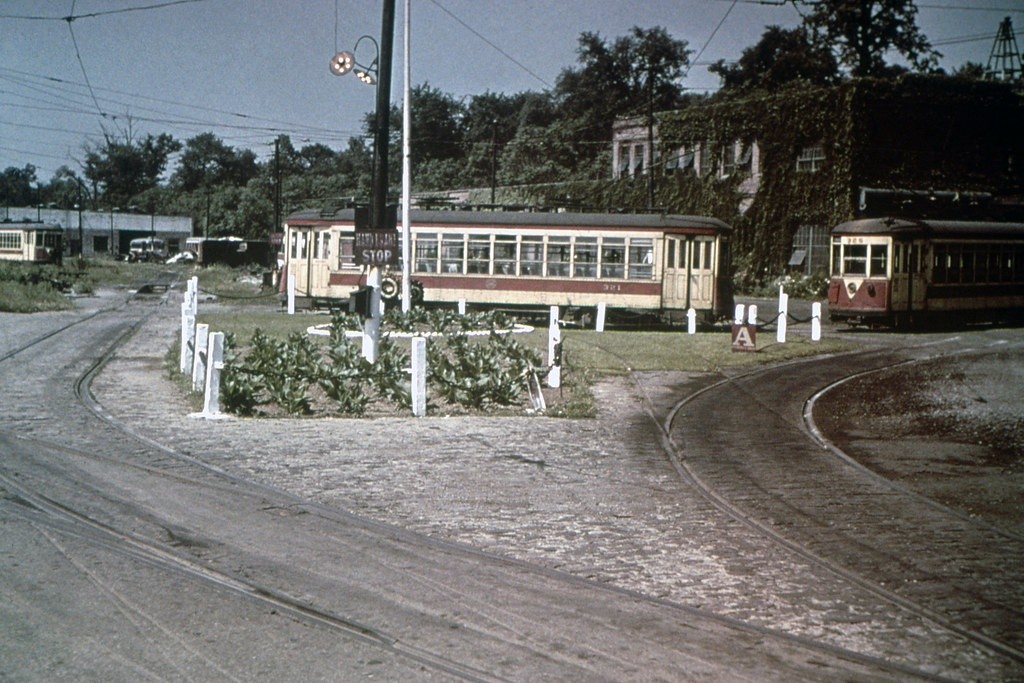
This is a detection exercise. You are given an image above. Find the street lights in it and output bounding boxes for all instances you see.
[330,1,403,367]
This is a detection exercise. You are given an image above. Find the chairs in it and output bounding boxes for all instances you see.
[467,264,622,278]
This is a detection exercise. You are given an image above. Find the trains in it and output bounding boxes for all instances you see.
[0,218,64,266]
[274,210,730,329]
[827,211,1022,330]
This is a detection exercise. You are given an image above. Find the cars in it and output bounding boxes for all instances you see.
[130,238,169,258]
